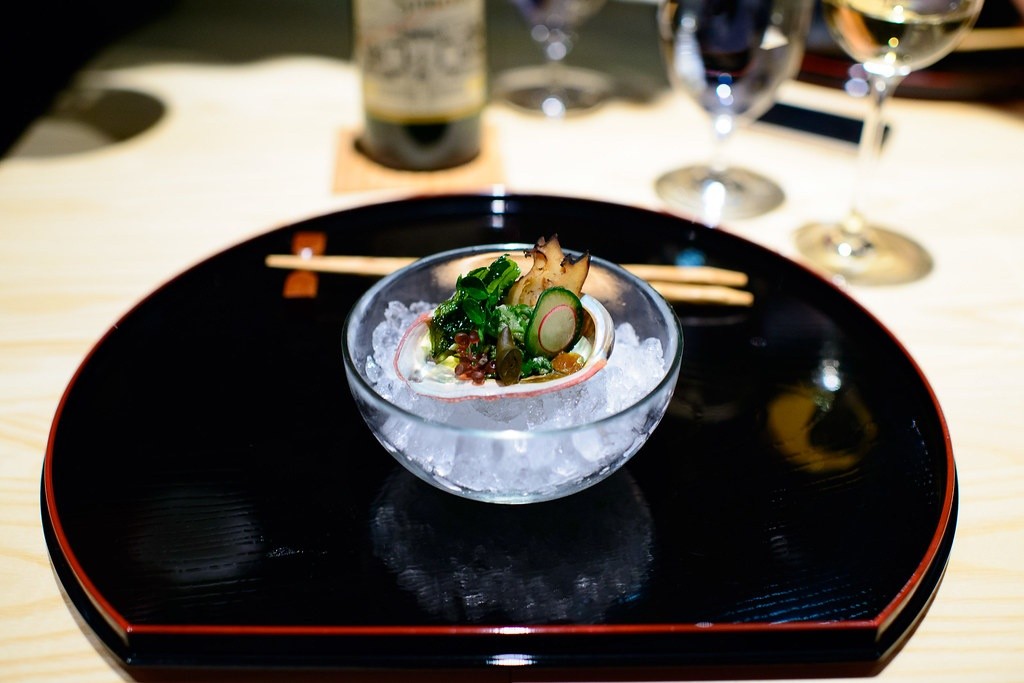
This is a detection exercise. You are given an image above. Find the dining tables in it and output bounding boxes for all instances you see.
[0,23,1024,683]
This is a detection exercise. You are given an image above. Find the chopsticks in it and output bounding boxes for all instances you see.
[265,254,755,306]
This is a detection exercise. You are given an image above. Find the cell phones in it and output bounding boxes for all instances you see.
[751,99,892,162]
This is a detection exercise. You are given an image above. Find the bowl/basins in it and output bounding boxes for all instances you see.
[341,244,683,503]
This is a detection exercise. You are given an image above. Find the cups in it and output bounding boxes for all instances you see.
[349,0,493,170]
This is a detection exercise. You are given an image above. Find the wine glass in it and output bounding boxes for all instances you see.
[650,0,812,218]
[514,0,606,116]
[791,0,986,290]
